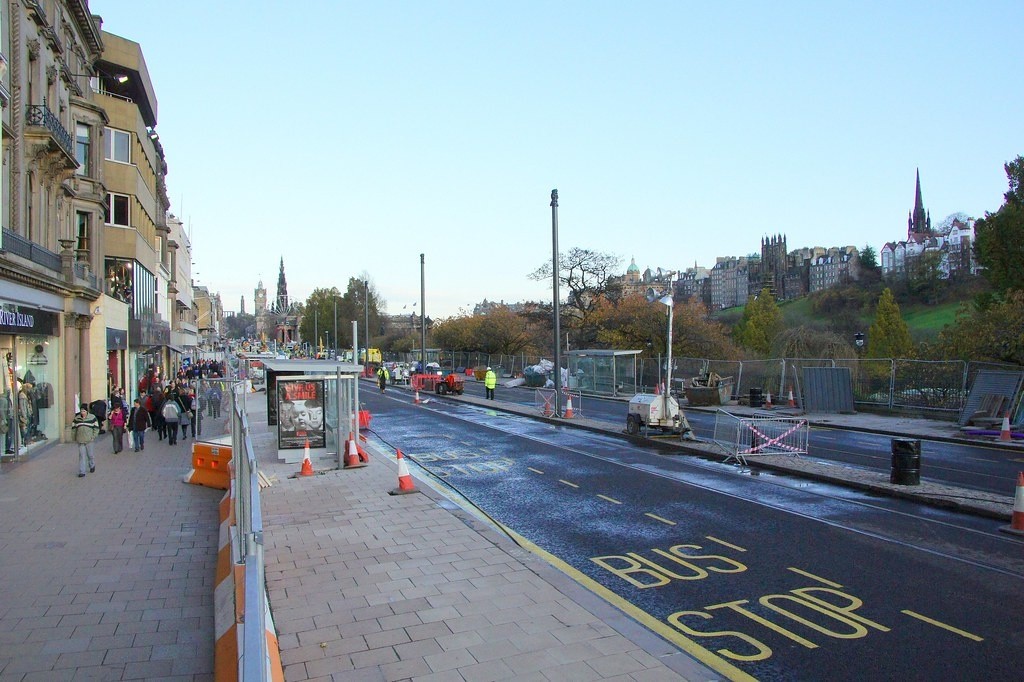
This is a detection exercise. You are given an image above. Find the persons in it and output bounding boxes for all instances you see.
[109,404,127,454]
[0,388,14,454]
[113,287,123,301]
[18,383,39,445]
[485,367,496,400]
[279,400,323,430]
[111,357,226,445]
[72,403,99,477]
[127,399,151,452]
[377,368,389,394]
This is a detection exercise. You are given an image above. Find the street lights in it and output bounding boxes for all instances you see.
[854,331,867,393]
[643,268,674,418]
[325,331,329,351]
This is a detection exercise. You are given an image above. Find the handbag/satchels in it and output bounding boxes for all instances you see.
[108,425,114,431]
[127,431,133,449]
[376,381,380,387]
[186,411,193,419]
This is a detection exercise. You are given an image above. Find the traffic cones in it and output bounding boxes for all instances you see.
[562,394,575,418]
[345,432,368,468]
[661,377,666,391]
[995,411,1016,443]
[562,378,568,392]
[295,438,319,479]
[761,391,774,410]
[654,384,659,394]
[785,386,798,408]
[997,472,1024,538]
[392,448,421,495]
[413,387,423,404]
[543,396,554,416]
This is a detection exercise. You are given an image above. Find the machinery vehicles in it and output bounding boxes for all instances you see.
[435,374,464,395]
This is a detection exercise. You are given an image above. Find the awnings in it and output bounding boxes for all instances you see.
[142,345,162,355]
[166,345,185,354]
[197,347,206,353]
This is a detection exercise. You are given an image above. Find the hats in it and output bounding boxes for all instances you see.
[487,367,491,370]
[80,404,87,411]
[382,367,386,371]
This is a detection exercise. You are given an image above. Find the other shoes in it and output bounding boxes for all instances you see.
[79,474,85,477]
[381,389,385,394]
[90,466,95,473]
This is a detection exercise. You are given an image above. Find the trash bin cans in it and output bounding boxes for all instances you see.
[891,437,921,485]
[473,364,505,380]
[524,370,555,386]
[750,388,762,407]
